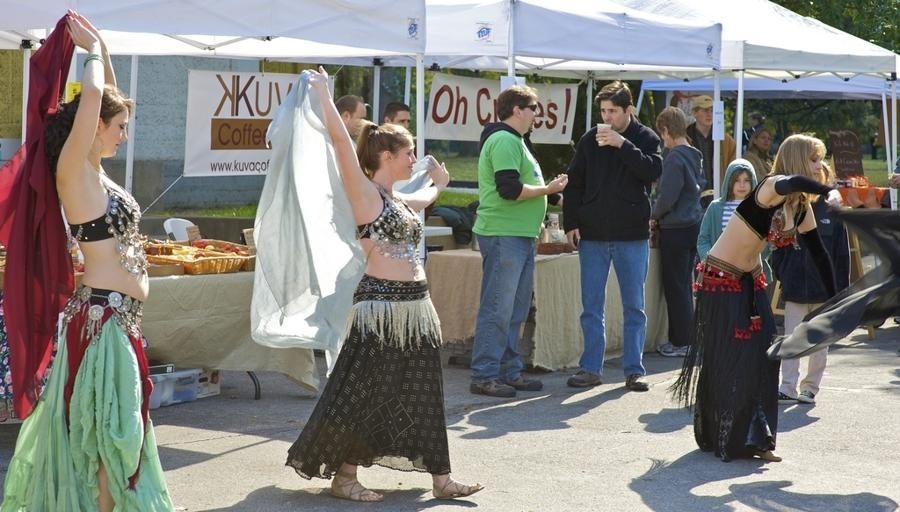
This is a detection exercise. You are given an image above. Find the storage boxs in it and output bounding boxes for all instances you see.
[197,367,221,399]
[149,366,203,411]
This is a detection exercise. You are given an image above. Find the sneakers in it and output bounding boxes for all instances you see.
[798,392,815,403]
[472,376,516,398]
[626,375,648,391]
[503,373,544,391]
[655,341,692,357]
[777,393,797,405]
[567,371,601,387]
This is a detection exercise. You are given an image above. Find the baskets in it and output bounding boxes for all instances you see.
[145,239,257,276]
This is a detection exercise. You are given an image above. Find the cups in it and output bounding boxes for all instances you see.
[541,213,561,244]
[596,123,613,144]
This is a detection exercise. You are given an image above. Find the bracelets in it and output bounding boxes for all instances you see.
[84,54,105,67]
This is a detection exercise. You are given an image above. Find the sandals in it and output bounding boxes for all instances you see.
[331,469,383,503]
[434,477,482,501]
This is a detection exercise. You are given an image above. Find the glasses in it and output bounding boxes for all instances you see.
[520,103,536,112]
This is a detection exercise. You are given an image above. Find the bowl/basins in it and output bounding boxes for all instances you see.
[839,185,884,206]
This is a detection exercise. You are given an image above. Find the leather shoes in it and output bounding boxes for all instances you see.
[758,451,780,461]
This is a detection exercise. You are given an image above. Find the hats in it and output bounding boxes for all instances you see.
[692,96,715,108]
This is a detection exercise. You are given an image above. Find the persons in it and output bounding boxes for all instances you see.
[0,9,173,512]
[697,158,773,285]
[650,107,703,357]
[888,158,900,188]
[871,133,878,159]
[524,132,564,225]
[334,95,367,150]
[771,162,850,404]
[743,125,773,183]
[562,80,663,391]
[470,85,569,397]
[285,65,486,502]
[685,95,736,208]
[383,102,411,130]
[665,133,843,461]
[742,112,765,150]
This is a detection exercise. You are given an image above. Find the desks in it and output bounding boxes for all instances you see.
[426,247,669,373]
[142,270,318,401]
